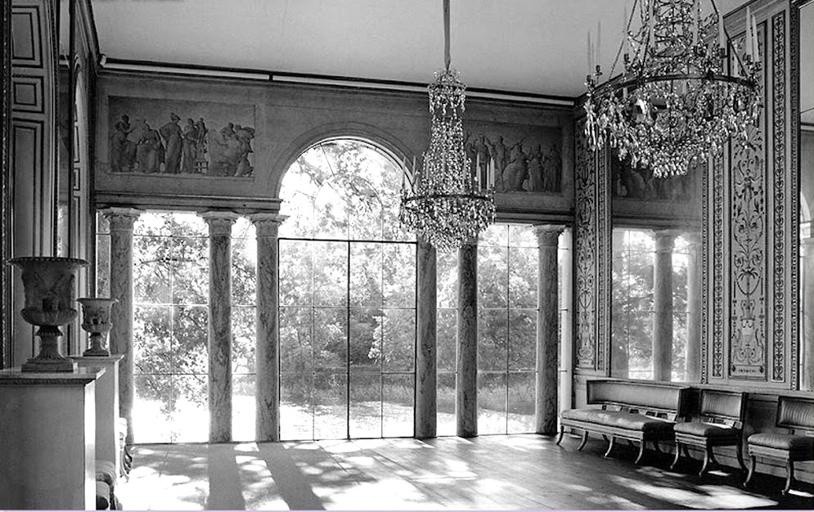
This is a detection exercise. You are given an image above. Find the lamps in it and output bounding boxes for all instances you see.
[396,1,499,255]
[581,2,765,182]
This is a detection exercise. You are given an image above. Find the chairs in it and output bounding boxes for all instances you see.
[743,394,814,495]
[668,387,750,479]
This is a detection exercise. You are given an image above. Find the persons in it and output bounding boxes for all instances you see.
[110,110,257,178]
[462,125,565,194]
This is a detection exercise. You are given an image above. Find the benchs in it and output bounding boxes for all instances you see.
[556,379,696,467]
